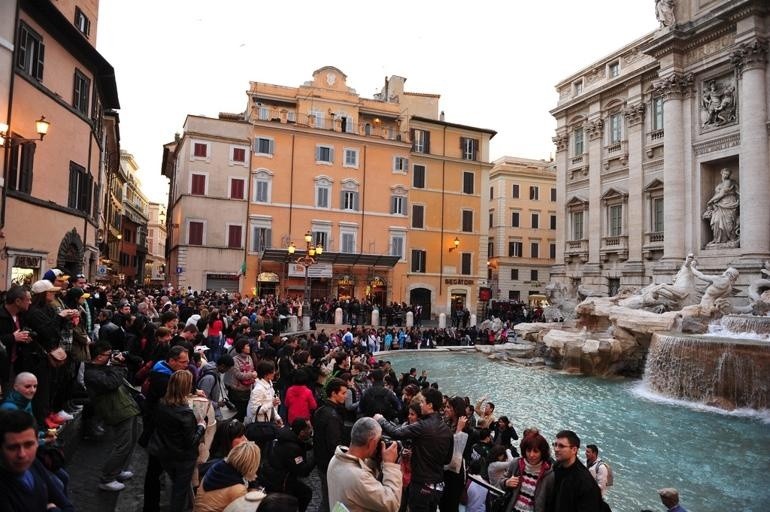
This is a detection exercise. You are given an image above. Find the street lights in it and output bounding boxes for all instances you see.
[0,114,50,149]
[286,228,324,320]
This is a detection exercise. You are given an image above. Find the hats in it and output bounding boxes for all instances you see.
[30,279,61,295]
[68,285,91,300]
[656,486,681,500]
[43,266,69,282]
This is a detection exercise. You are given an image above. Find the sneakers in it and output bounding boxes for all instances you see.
[98,479,128,492]
[113,470,134,481]
[41,401,83,433]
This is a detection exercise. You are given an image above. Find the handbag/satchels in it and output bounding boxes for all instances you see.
[242,402,282,442]
[46,347,68,368]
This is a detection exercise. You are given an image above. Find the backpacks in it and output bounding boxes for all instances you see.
[595,459,615,488]
[235,354,256,387]
[320,369,343,404]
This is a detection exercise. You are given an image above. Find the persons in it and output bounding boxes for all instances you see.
[714,86,735,125]
[667,262,738,315]
[705,166,740,242]
[701,82,724,125]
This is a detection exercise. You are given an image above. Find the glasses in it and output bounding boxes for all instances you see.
[75,274,86,278]
[552,441,576,449]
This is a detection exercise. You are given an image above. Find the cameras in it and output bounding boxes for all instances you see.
[112,350,128,360]
[221,397,236,410]
[377,438,403,453]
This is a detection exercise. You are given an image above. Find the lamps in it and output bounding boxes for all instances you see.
[448,236,461,253]
[1,113,51,149]
